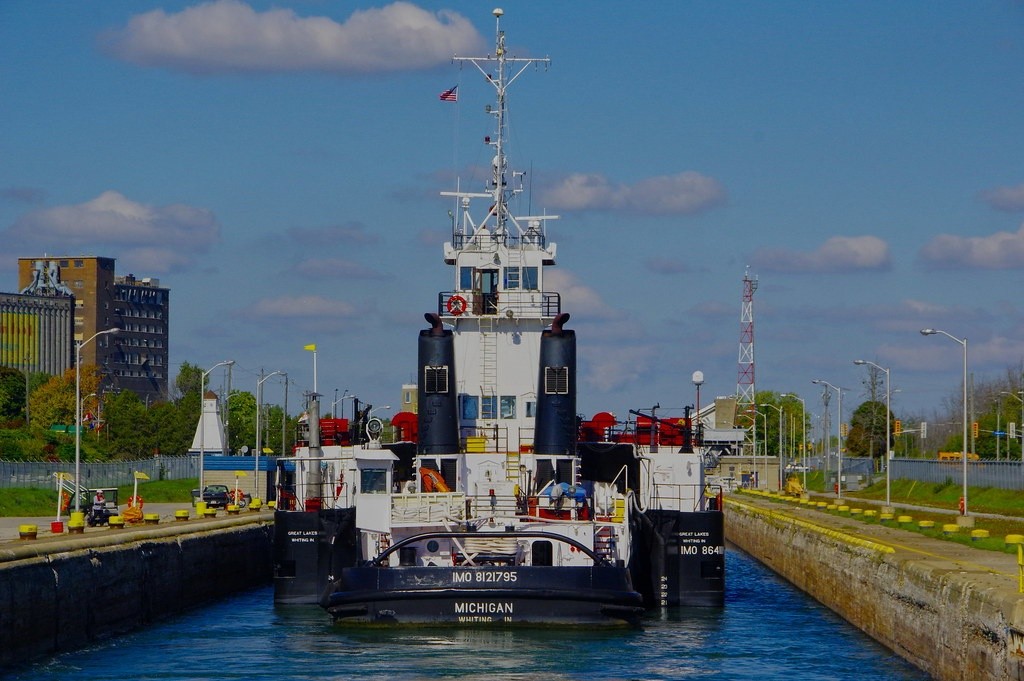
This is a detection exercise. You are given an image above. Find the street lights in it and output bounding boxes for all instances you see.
[196,361,235,512]
[370,406,391,420]
[332,395,356,419]
[920,328,975,528]
[737,394,810,500]
[71,328,120,520]
[222,394,238,426]
[812,380,845,505]
[1001,392,1024,461]
[853,359,894,514]
[81,394,96,425]
[880,390,902,472]
[252,372,288,505]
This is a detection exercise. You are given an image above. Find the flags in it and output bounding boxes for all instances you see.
[440,86,457,102]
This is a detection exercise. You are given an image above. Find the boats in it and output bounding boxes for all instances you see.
[272,8,725,628]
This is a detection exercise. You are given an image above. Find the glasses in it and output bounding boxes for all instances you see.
[99,493,102,494]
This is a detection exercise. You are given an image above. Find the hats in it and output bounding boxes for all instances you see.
[97,490,103,493]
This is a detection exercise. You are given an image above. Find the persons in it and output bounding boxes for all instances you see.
[89,490,108,527]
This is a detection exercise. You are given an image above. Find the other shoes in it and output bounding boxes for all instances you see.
[101,525,106,527]
[87,524,91,528]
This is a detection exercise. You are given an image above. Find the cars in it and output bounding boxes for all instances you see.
[203,484,231,509]
[784,463,809,472]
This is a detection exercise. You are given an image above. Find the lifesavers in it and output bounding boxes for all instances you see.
[230,488,244,501]
[60,490,70,510]
[959,497,964,515]
[447,295,467,315]
[127,495,144,510]
[835,482,838,494]
